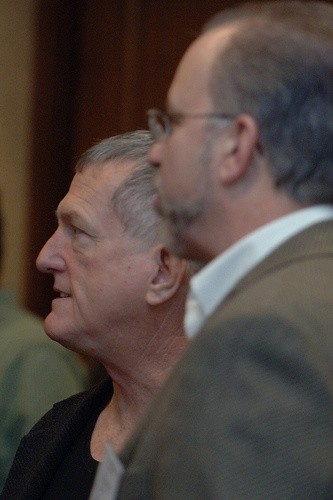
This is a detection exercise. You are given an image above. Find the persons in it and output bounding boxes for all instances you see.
[94,2,332,500]
[0,132,208,499]
[0,214,90,497]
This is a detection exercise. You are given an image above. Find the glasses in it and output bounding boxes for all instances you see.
[145,110,266,158]
